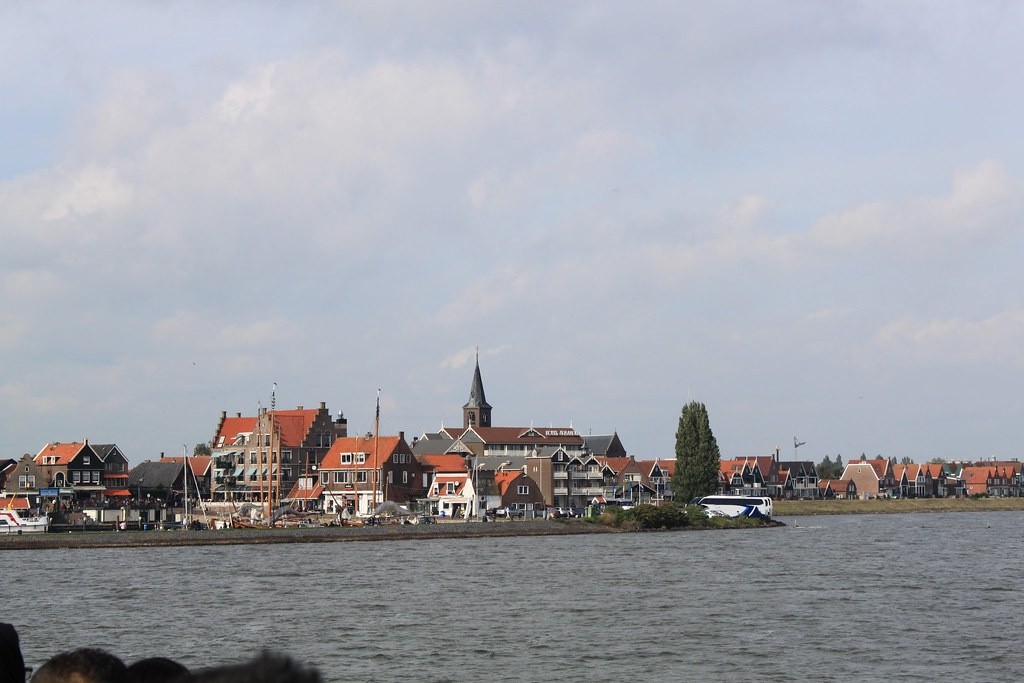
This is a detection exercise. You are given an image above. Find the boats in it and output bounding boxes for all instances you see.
[0,493,53,534]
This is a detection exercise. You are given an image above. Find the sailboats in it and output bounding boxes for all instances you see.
[230,383,381,529]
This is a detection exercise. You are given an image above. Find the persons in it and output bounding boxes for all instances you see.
[119,521,127,531]
[0,622,325,683]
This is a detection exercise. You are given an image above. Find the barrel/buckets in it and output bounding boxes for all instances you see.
[441,511,445,517]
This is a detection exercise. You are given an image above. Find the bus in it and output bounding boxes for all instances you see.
[689,493,773,519]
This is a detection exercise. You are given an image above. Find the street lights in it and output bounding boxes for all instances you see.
[475,454,486,523]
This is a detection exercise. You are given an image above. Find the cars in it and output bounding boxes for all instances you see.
[554,506,585,518]
[486,505,524,518]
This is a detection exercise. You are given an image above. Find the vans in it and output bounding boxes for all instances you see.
[604,496,637,511]
